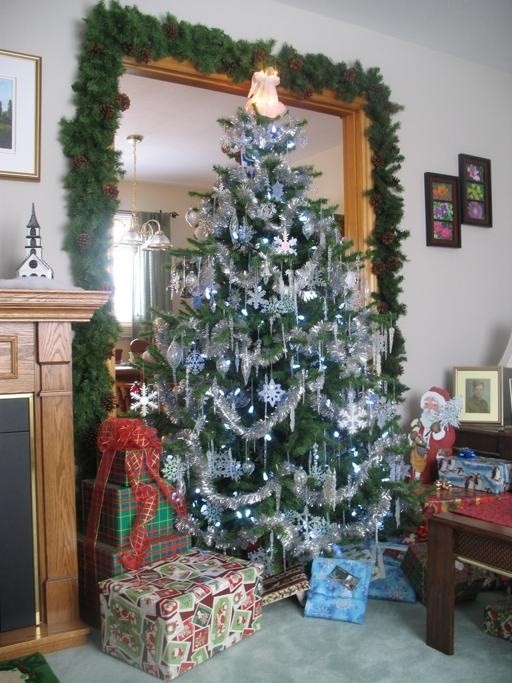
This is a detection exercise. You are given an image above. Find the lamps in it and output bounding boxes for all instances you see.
[244,66,288,119]
[109,134,174,252]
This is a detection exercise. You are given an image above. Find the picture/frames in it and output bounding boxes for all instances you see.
[424,151,491,247]
[455,365,512,425]
[0,49,44,181]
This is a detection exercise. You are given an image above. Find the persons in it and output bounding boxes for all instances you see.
[465,378,491,410]
[406,385,457,481]
